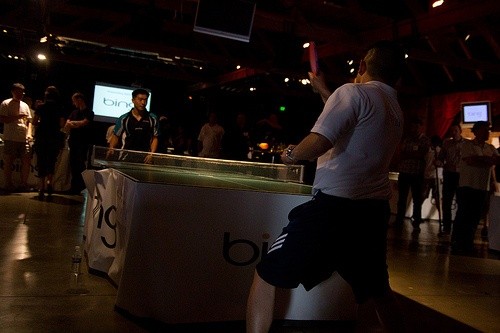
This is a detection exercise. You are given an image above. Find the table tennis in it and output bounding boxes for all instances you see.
[260,142,268,148]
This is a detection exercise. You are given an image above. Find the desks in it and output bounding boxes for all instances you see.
[388,171,485,224]
[81,158,363,332]
[0,143,73,191]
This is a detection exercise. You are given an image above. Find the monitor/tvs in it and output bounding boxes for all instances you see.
[91,81,153,124]
[460,101,492,128]
[194,0,257,44]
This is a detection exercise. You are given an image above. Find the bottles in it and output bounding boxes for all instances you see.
[71,246,81,274]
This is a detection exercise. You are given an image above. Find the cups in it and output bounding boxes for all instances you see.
[22,112,28,121]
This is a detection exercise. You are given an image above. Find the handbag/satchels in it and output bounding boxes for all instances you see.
[31,124,45,153]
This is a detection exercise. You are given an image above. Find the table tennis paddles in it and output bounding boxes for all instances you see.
[308,41,319,94]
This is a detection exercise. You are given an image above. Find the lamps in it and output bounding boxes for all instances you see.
[37,43,50,60]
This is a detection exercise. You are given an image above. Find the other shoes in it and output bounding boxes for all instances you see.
[481,228,489,241]
[442,227,452,233]
[408,215,421,233]
[46,196,53,202]
[35,193,44,201]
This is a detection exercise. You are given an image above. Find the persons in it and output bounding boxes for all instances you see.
[31,85,65,202]
[65,92,96,195]
[392,115,429,235]
[448,121,499,257]
[220,112,251,159]
[432,123,466,236]
[0,82,33,192]
[196,113,225,158]
[245,38,406,333]
[24,94,43,129]
[425,138,445,230]
[104,88,159,164]
[53,113,77,185]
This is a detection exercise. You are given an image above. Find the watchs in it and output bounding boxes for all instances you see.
[286,146,298,163]
